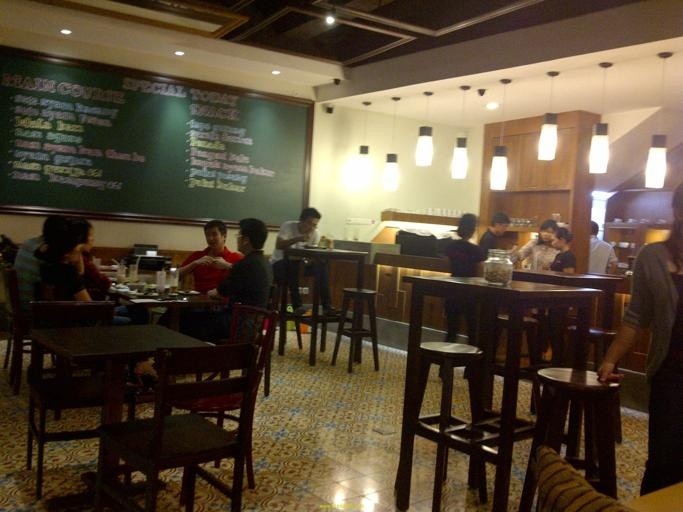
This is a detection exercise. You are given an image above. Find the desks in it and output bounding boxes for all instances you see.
[277,247,369,367]
[394,275,603,512]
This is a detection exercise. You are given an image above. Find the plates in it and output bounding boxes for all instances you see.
[135,292,159,297]
[179,290,201,295]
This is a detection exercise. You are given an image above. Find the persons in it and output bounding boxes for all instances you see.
[588,221,617,274]
[41,222,91,302]
[15,215,65,310]
[0,234,18,265]
[551,227,576,273]
[155,219,244,325]
[479,212,511,252]
[271,208,342,316]
[438,213,488,379]
[67,217,111,301]
[597,183,683,496]
[509,219,561,271]
[180,218,273,342]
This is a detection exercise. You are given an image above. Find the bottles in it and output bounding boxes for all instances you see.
[483,249,512,287]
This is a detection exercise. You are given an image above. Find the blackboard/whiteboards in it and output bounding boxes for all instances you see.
[0,44,316,231]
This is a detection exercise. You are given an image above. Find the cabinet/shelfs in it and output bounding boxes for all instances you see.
[478,111,599,275]
[372,209,464,244]
[601,189,679,278]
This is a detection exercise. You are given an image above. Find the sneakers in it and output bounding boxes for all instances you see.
[323,307,342,316]
[295,307,306,317]
[126,381,138,388]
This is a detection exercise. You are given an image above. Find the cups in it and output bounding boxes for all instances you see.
[116,264,127,283]
[156,270,169,299]
[130,263,139,284]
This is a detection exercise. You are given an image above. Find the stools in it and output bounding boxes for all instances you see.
[395,339,487,512]
[331,287,379,374]
[495,315,543,415]
[563,326,618,471]
[518,365,621,510]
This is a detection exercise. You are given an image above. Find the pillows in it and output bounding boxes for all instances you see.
[528,446,638,512]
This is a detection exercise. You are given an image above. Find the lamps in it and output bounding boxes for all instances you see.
[645,52,674,189]
[589,63,613,175]
[450,85,470,181]
[490,78,511,192]
[536,71,561,161]
[343,101,373,192]
[415,90,434,167]
[381,96,401,196]
